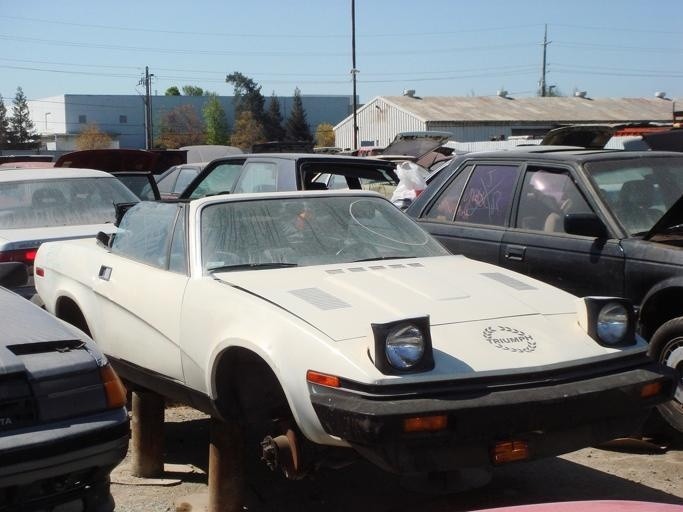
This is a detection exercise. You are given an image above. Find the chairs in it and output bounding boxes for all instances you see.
[32,188,66,209]
[618,179,664,232]
[306,181,329,191]
